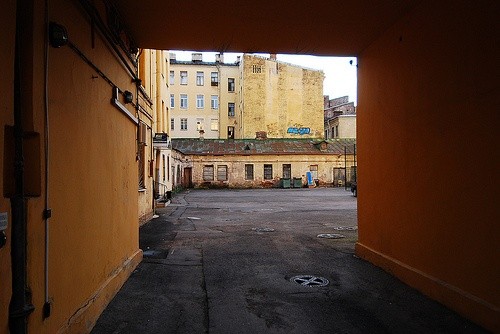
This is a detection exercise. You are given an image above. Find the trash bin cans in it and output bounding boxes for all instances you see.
[293,176,303,188]
[280,177,291,189]
